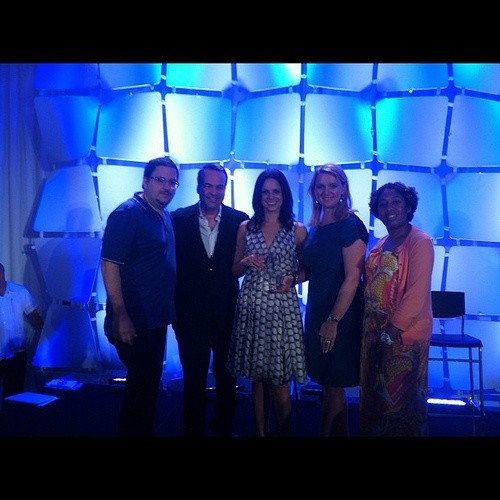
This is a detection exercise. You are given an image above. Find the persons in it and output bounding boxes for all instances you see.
[357,181,434,436]
[0,262,50,395]
[101,157,179,436]
[231,168,308,436]
[174,161,250,436]
[297,163,370,436]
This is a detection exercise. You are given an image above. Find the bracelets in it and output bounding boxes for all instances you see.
[287,271,298,288]
[328,314,341,325]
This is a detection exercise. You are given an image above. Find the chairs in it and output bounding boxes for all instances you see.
[424,291,484,410]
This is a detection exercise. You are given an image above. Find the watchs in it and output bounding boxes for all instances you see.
[379,330,393,346]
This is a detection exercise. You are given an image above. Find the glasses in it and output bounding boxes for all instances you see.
[148,175,179,189]
